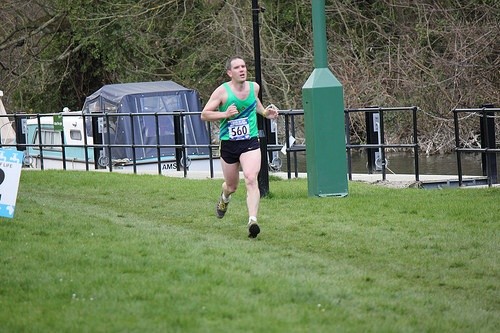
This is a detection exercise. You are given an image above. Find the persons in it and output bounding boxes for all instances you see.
[200,56,279,239]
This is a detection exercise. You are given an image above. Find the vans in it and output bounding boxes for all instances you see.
[22,116,93,161]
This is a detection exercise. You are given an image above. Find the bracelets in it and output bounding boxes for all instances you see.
[262,109,267,117]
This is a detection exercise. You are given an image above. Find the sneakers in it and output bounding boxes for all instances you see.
[216,192,230,219]
[248,221,260,238]
